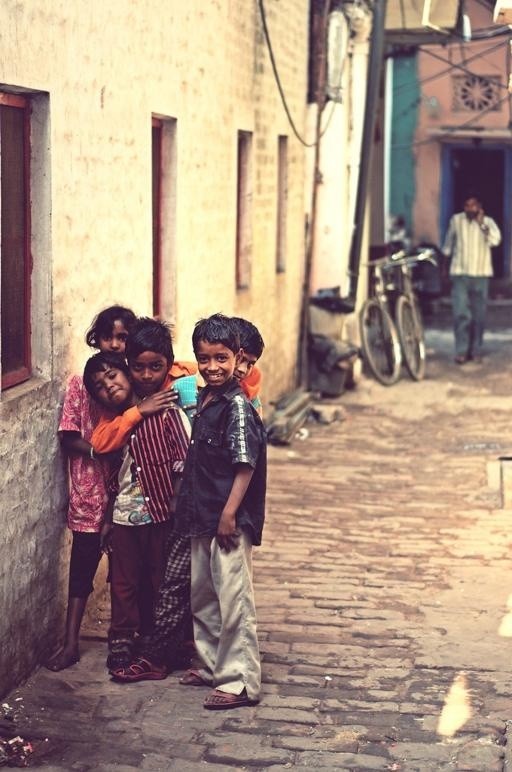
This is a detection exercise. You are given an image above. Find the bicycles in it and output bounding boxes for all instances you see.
[358,247,404,387]
[383,247,439,382]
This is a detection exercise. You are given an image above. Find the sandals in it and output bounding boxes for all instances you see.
[106,637,258,709]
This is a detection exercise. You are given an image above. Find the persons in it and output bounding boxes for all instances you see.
[79,350,193,670]
[171,312,271,711]
[434,190,506,366]
[44,305,138,675]
